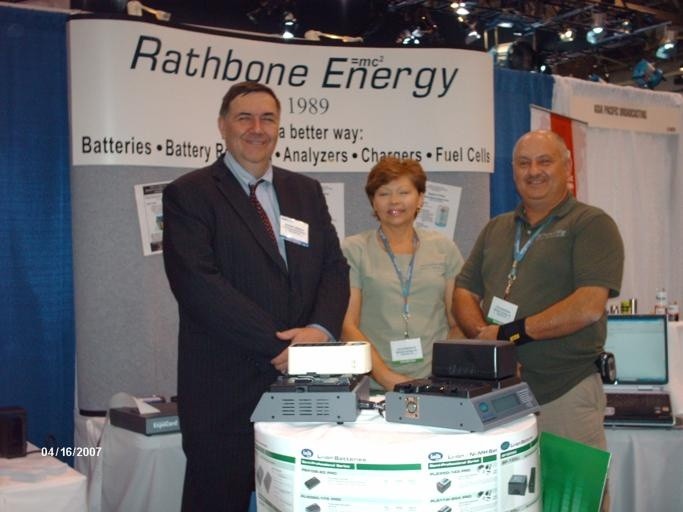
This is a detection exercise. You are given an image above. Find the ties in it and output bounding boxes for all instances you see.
[248,180,283,261]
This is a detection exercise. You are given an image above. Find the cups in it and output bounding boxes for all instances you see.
[609,296,637,316]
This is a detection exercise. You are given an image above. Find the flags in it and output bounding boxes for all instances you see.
[530,105,588,206]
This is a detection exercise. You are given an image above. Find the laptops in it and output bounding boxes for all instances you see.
[602,315,675,427]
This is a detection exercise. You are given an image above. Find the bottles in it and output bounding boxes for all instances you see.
[653,286,680,322]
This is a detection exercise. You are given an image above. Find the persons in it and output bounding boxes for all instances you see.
[341,156,465,397]
[161,80,352,512]
[449,129,625,512]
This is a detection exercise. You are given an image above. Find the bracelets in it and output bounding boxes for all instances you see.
[497,317,534,347]
